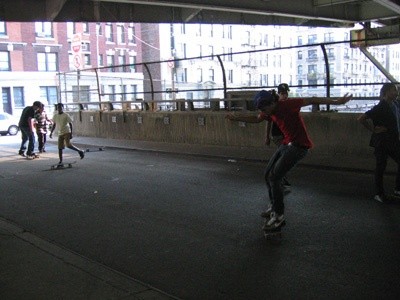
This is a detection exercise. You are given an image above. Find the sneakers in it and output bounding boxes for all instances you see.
[27,153,35,156]
[52,162,64,168]
[373,195,383,203]
[262,211,287,231]
[79,150,85,159]
[391,191,400,199]
[18,153,26,157]
[259,204,272,217]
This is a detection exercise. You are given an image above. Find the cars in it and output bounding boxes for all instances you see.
[0,113,20,136]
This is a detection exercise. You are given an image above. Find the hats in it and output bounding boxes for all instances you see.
[34,101,42,108]
[255,90,272,108]
[278,83,290,93]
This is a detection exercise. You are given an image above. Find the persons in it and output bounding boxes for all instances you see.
[19,101,41,157]
[356,82,400,203]
[266,83,292,186]
[49,103,85,165]
[225,90,354,231]
[33,104,53,152]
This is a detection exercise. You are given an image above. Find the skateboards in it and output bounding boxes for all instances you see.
[27,154,40,160]
[47,161,77,169]
[264,202,282,240]
[85,148,104,152]
